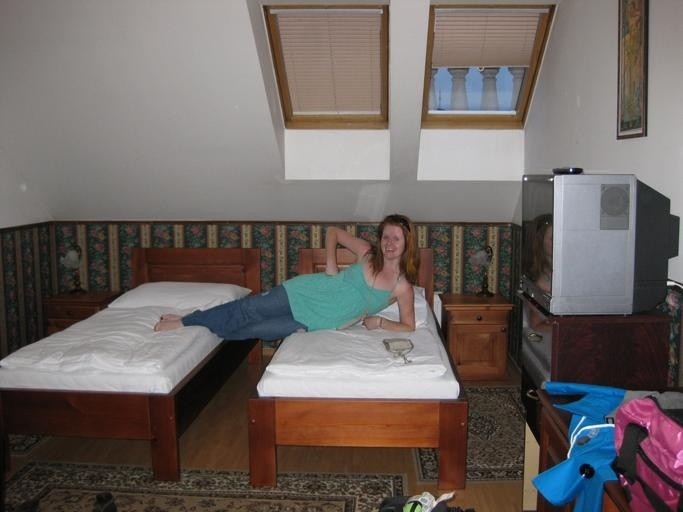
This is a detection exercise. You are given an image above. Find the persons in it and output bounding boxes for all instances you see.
[155,214,420,340]
[527,214,553,297]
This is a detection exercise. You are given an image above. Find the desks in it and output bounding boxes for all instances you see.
[535,386,682,512]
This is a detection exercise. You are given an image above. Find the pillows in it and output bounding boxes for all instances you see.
[371,280,430,328]
[109,281,252,311]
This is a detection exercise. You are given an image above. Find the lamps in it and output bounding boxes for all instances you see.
[60,244,89,298]
[475,243,493,297]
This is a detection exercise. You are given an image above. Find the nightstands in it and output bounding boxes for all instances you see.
[42,290,118,338]
[439,293,515,381]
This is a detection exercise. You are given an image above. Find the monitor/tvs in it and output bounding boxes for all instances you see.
[521,173,680,316]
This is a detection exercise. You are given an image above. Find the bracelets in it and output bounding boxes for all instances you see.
[381,317,384,330]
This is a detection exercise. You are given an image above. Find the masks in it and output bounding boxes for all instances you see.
[384,338,413,363]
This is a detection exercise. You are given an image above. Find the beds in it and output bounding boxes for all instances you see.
[1,248,261,481]
[245,246,467,488]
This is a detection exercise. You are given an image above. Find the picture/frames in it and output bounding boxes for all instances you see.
[616,16,648,140]
